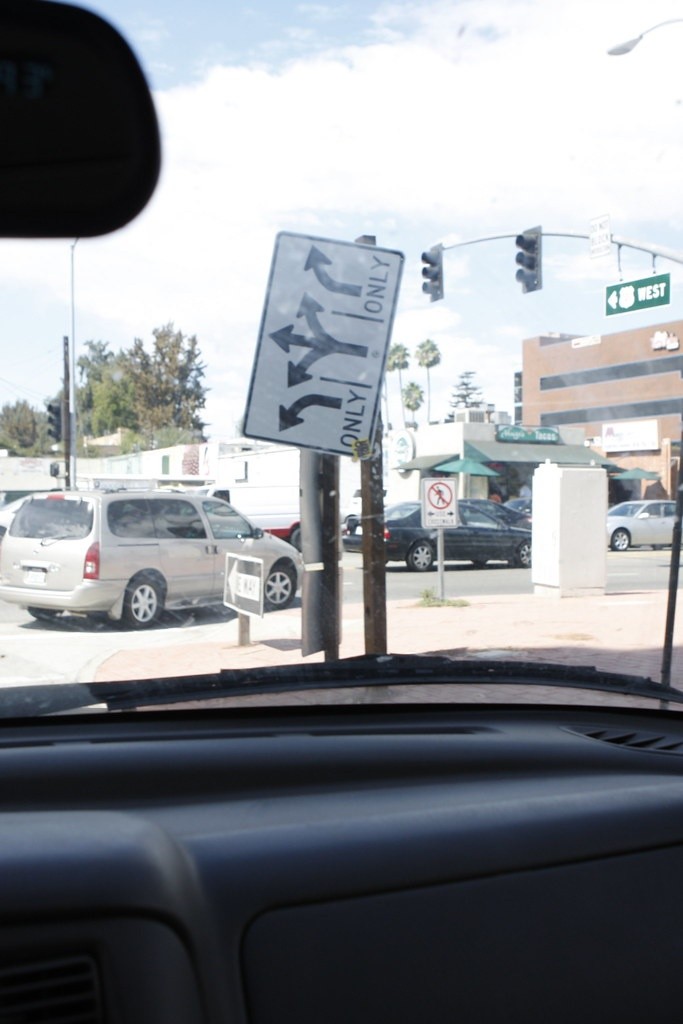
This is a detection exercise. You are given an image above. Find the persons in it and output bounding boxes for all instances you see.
[489,480,531,502]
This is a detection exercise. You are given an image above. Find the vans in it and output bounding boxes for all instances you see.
[161,482,302,553]
[0,489,304,630]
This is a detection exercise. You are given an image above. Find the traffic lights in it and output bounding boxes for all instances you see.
[421,243,444,301]
[516,226,543,293]
[47,404,61,442]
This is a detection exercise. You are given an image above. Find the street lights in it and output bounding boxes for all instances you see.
[607,17,683,56]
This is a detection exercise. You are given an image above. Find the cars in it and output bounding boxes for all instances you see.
[459,499,532,530]
[503,498,532,516]
[608,500,683,552]
[342,503,532,572]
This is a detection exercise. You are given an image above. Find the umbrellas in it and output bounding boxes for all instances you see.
[434,457,500,498]
[612,468,661,499]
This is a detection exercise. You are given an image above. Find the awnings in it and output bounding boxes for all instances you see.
[464,438,617,473]
[394,453,459,474]
